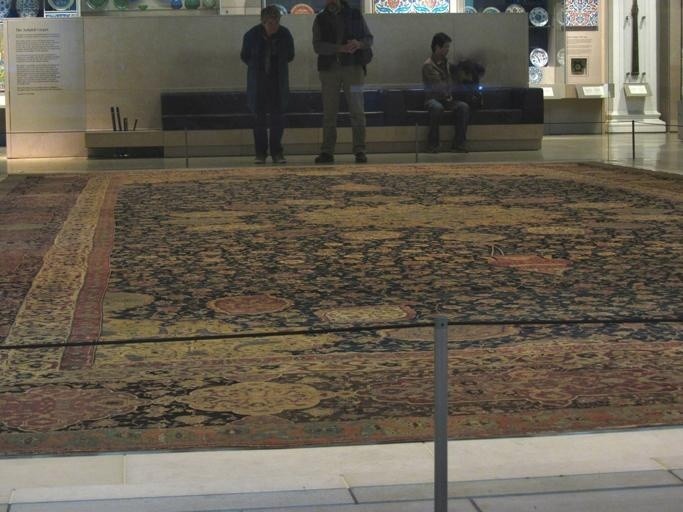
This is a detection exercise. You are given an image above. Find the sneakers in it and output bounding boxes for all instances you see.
[426,139,439,154]
[254,158,266,164]
[272,155,288,164]
[450,143,468,154]
[354,152,367,163]
[314,152,335,164]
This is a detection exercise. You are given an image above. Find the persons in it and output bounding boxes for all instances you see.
[311,0,374,163]
[420,33,472,154]
[238,7,294,164]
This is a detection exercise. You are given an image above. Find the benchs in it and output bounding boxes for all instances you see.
[157,88,544,149]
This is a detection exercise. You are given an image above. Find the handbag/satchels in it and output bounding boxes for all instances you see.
[353,46,373,66]
